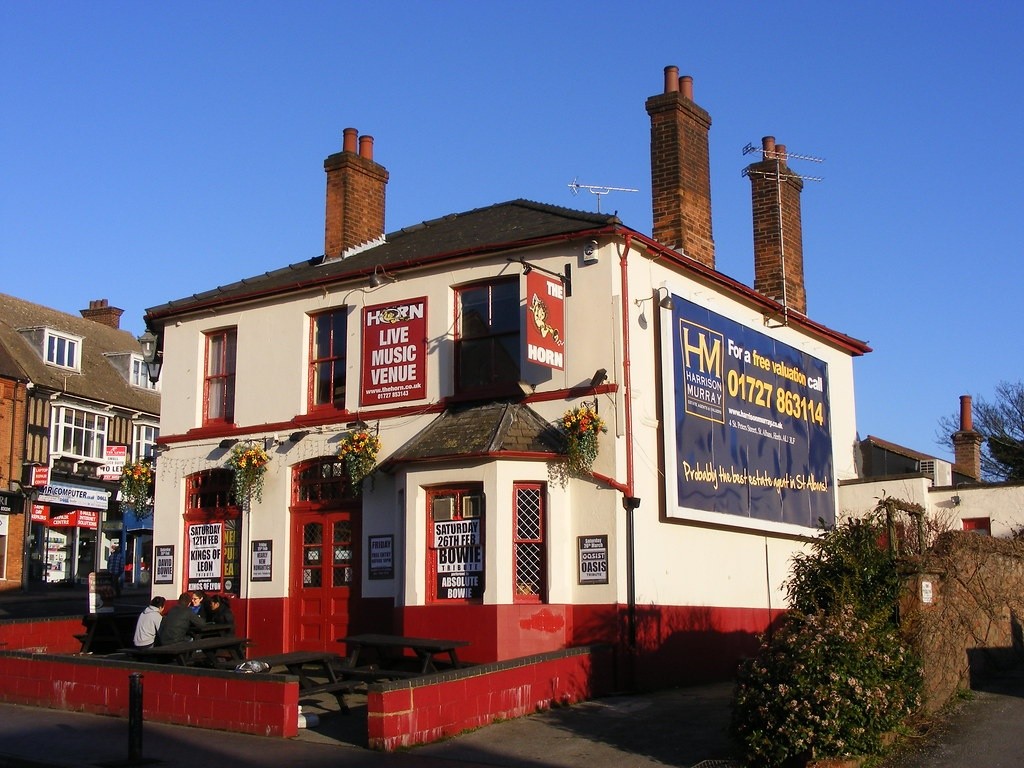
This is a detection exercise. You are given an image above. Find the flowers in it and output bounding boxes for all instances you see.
[336,430,382,500]
[550,407,609,492]
[223,444,273,515]
[118,460,155,523]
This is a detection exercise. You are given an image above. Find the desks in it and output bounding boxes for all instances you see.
[82,614,140,653]
[193,624,239,662]
[215,651,351,716]
[336,633,471,673]
[144,635,253,667]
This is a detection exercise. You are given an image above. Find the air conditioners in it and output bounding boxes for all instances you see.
[920,459,952,487]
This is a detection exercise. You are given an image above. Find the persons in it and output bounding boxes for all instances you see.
[107,546,124,599]
[133,596,166,650]
[189,591,207,614]
[201,594,235,662]
[160,592,206,666]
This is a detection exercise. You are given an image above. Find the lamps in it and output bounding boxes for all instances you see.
[348,421,368,430]
[142,351,163,385]
[219,439,239,449]
[289,431,306,442]
[369,263,398,289]
[137,327,158,363]
[635,286,673,310]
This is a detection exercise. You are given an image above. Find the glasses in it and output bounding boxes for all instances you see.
[191,598,199,601]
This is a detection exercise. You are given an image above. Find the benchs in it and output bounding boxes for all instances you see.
[117,648,146,656]
[300,678,364,698]
[333,667,424,680]
[385,656,481,669]
[80,633,134,644]
